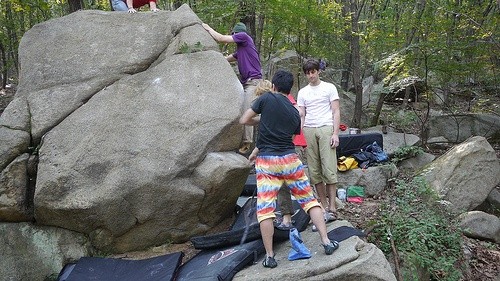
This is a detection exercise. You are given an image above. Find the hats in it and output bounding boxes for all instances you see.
[230,22,247,33]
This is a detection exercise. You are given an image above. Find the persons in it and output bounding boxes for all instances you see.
[200,20,263,155]
[110,0,160,14]
[238,70,339,268]
[297,60,339,221]
[318,57,330,71]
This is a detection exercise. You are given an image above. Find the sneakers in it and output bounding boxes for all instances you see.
[327,210,338,222]
[323,240,339,254]
[262,254,277,268]
[278,222,294,230]
[239,144,251,153]
